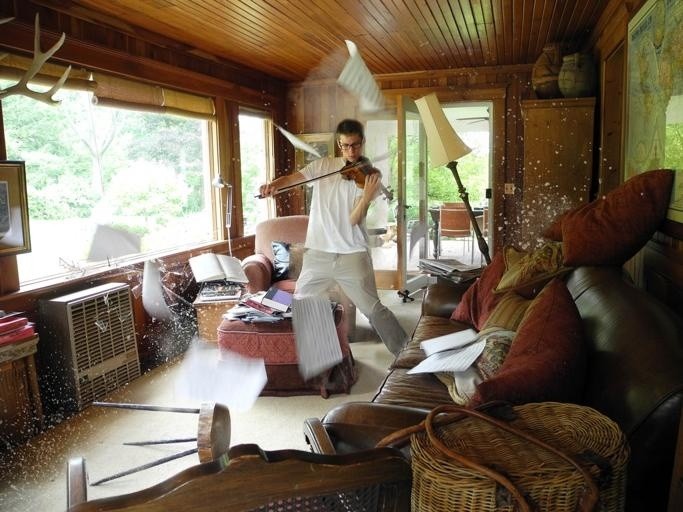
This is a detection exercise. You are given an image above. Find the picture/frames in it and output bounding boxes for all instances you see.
[2,161,33,255]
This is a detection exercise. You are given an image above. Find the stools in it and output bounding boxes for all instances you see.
[87,395,230,487]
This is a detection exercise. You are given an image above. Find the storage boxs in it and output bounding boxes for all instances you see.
[192,292,244,342]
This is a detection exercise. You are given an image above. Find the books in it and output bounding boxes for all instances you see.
[243,285,295,318]
[188,252,250,284]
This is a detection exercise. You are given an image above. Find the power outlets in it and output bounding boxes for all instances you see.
[505,184,515,194]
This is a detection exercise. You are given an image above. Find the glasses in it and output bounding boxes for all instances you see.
[338,140,362,150]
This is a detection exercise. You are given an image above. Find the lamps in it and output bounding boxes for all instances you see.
[212,174,232,257]
[414,92,490,263]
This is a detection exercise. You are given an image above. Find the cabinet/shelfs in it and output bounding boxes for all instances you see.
[0,334,49,438]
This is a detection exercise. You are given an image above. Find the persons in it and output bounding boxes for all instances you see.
[259,119,411,356]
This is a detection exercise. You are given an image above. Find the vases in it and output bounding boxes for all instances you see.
[531,46,560,99]
[560,53,592,96]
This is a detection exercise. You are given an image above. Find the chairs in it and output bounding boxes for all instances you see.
[64,416,409,512]
[443,202,470,252]
[472,209,489,266]
[438,208,473,257]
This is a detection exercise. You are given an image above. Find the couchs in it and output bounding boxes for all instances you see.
[238,216,355,342]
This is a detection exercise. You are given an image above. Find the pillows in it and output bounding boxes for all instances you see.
[470,327,515,380]
[559,169,677,266]
[481,294,533,331]
[322,267,682,512]
[450,252,504,329]
[503,245,526,266]
[479,278,583,405]
[492,240,569,291]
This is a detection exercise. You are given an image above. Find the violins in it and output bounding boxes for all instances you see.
[340,157,393,201]
